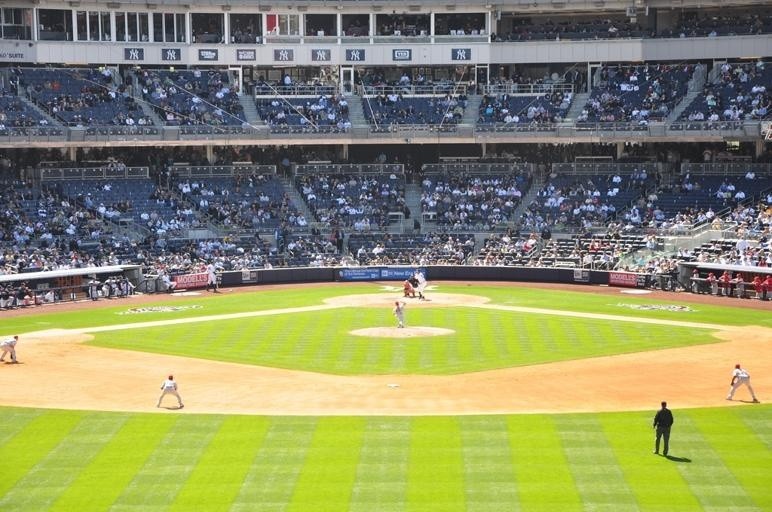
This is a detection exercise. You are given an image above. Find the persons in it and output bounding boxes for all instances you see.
[392,300,408,328]
[1,335,20,364]
[726,363,760,404]
[155,374,185,408]
[651,400,673,456]
[1,0,771,313]
